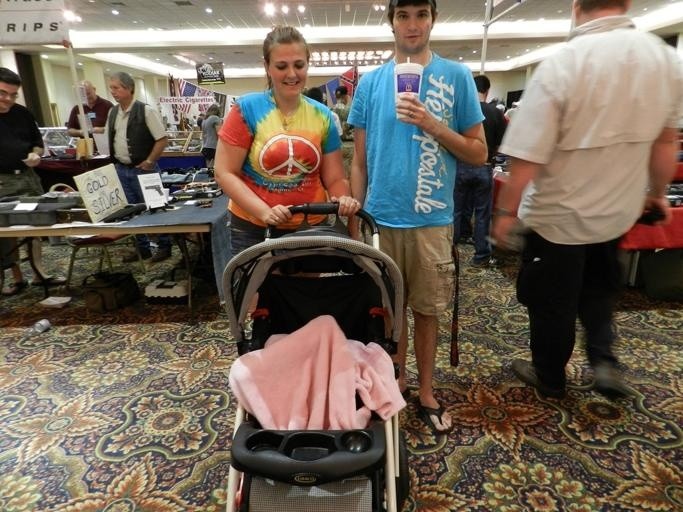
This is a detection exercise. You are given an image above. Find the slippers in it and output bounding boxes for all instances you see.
[31,274,66,285]
[414,394,453,434]
[1,278,27,296]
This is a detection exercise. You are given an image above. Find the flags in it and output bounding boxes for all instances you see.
[341,66,359,97]
[179,78,239,121]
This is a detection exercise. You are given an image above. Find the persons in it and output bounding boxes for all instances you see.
[454,74,523,270]
[64,78,116,138]
[102,72,173,264]
[210,26,360,317]
[343,2,489,438]
[308,85,354,179]
[177,104,222,169]
[0,66,68,296]
[489,0,683,400]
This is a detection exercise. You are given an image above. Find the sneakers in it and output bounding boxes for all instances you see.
[472,254,505,268]
[151,246,170,262]
[458,234,475,245]
[123,248,151,262]
[509,358,567,398]
[593,361,629,400]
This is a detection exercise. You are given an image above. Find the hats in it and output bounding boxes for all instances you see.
[335,86,346,95]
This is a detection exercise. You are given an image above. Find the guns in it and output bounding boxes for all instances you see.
[145,185,163,196]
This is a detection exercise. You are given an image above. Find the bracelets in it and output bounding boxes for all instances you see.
[146,160,156,168]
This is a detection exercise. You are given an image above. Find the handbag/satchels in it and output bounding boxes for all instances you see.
[81,271,140,314]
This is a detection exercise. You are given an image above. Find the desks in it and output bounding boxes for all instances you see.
[493,170,683,289]
[0,172,231,317]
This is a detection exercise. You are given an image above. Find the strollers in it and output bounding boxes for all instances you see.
[216,206,412,508]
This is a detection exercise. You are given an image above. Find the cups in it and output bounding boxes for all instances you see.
[393,63,424,119]
